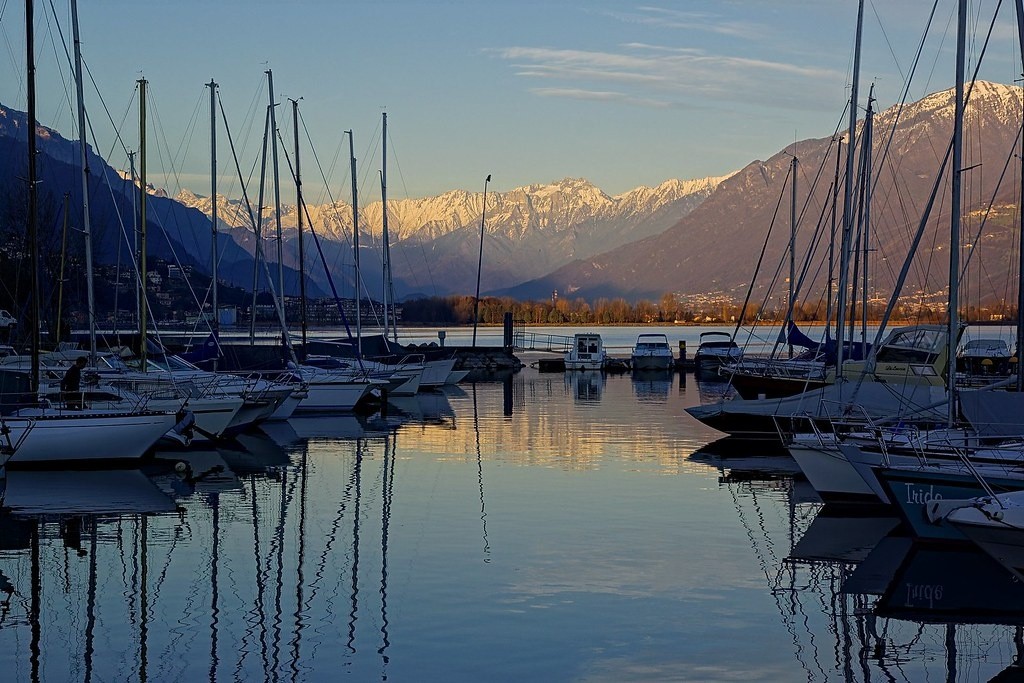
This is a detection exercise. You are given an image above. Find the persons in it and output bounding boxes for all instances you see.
[77,341,87,350]
[53,342,60,352]
[60,356,88,410]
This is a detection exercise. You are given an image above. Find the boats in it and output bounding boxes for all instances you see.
[695,332,745,370]
[630,333,675,371]
[564,331,605,371]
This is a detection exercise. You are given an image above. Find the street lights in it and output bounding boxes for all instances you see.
[472,173,492,349]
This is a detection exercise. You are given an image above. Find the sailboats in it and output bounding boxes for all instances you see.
[683,1,1023,588]
[0,1,470,464]
